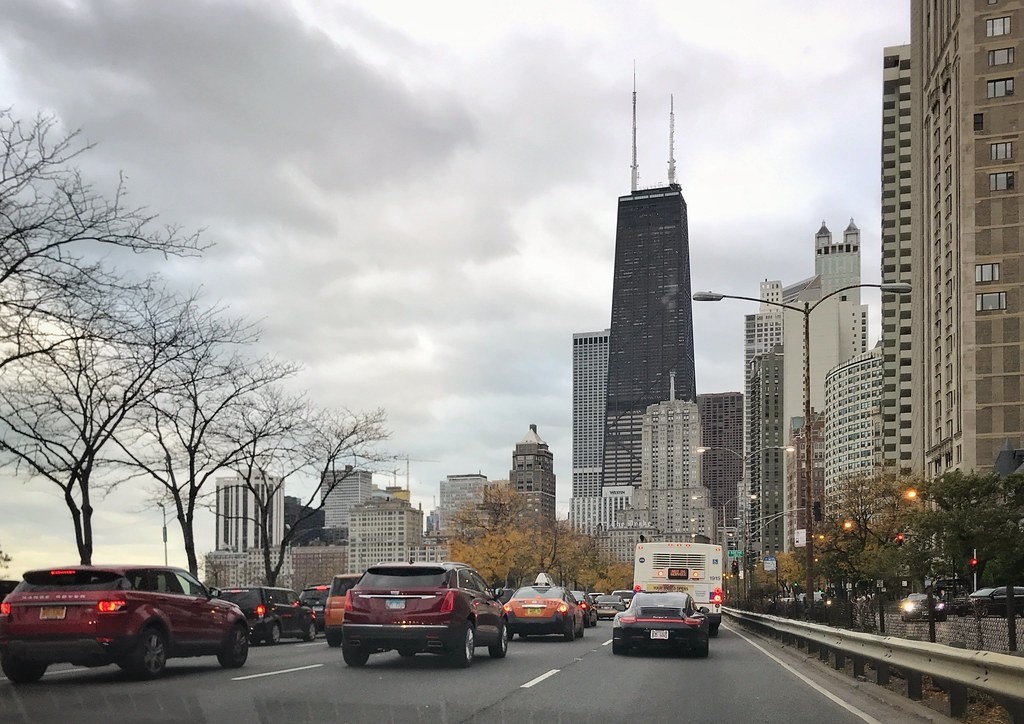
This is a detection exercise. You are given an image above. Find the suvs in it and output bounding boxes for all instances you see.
[950,586,1024,618]
[0,565,250,683]
[342,560,509,668]
[294,584,331,631]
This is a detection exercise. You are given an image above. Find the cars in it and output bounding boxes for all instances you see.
[492,588,515,604]
[611,590,635,609]
[218,587,318,646]
[570,591,598,627]
[612,589,709,658]
[595,596,629,620]
[502,573,585,641]
[900,594,947,622]
[323,574,364,647]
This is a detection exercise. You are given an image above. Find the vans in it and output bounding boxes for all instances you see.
[588,593,604,608]
[799,592,828,603]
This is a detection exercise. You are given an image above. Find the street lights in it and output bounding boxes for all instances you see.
[286,524,292,589]
[693,444,796,603]
[694,282,914,602]
[340,539,350,574]
[691,495,759,601]
[692,518,740,544]
[157,502,168,567]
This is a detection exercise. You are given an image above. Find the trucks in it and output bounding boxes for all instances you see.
[633,534,724,636]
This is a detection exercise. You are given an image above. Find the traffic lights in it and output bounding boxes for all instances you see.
[818,588,821,592]
[795,581,797,587]
[731,561,738,575]
[898,536,902,545]
[811,502,821,521]
[971,560,977,573]
